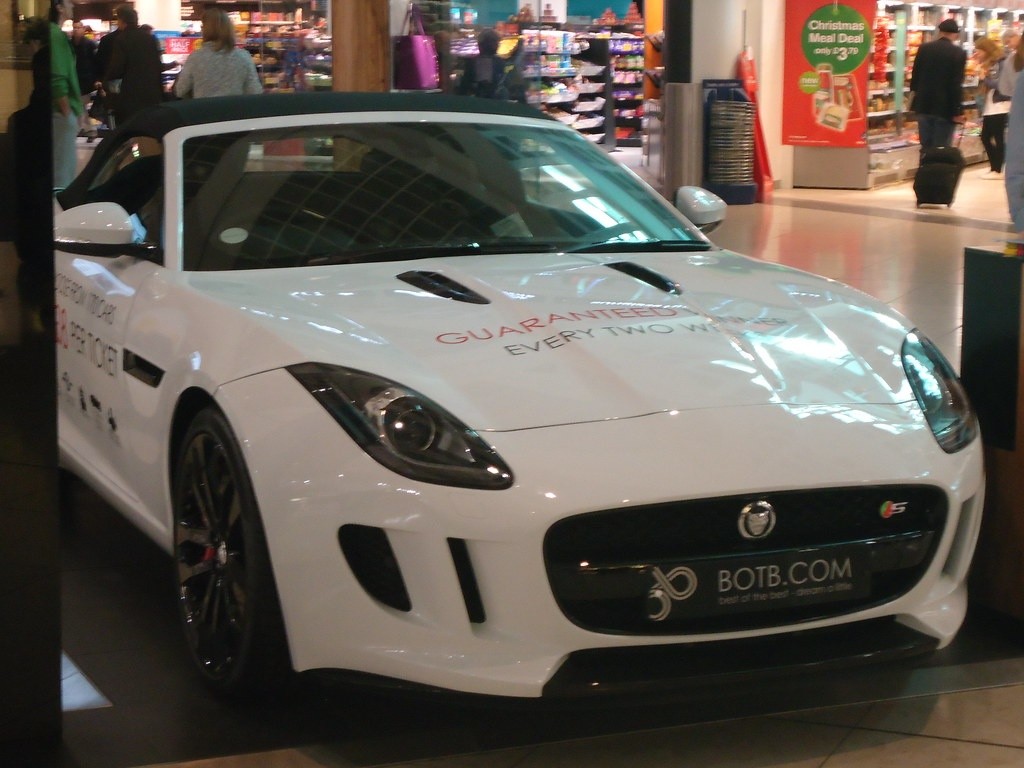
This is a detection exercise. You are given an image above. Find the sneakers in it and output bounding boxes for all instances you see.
[979,170,1004,180]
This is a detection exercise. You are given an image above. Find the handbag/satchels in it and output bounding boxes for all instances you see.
[392,5,439,89]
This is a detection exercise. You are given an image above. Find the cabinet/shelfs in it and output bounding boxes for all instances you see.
[791,3,1024,188]
[62,0,332,94]
[422,5,665,169]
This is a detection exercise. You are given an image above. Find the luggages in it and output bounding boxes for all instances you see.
[912,117,965,208]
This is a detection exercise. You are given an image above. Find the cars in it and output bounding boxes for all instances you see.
[54,89,988,723]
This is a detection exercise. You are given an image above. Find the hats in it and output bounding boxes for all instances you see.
[939,18,959,33]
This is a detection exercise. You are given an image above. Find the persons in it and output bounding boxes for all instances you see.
[971,36,1013,181]
[997,24,1024,238]
[0,0,169,207]
[172,8,264,100]
[457,29,507,97]
[906,18,967,161]
